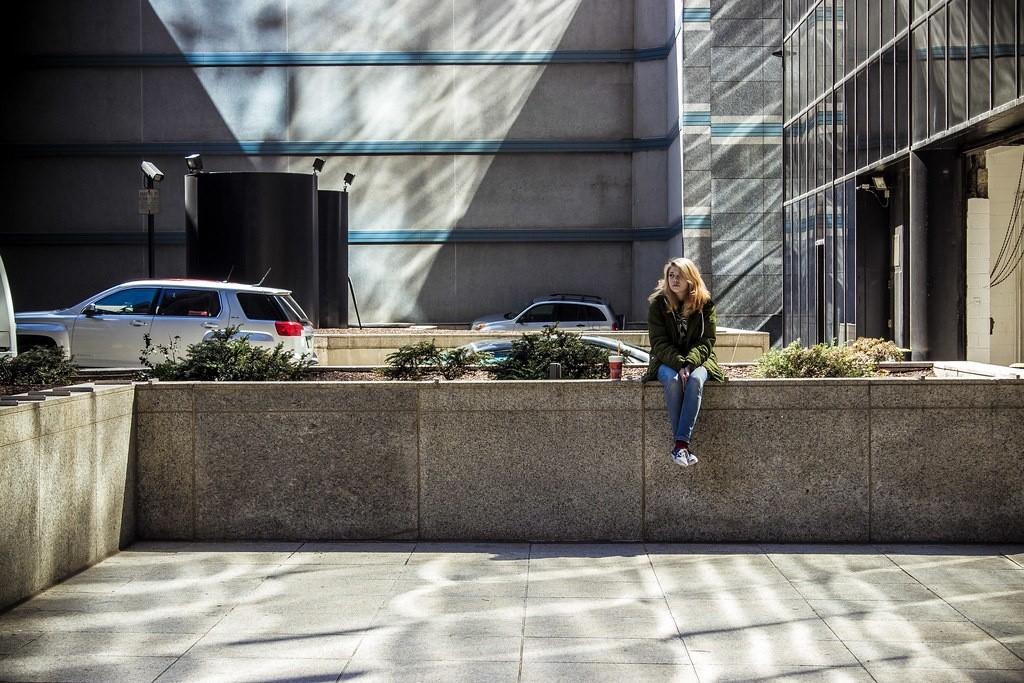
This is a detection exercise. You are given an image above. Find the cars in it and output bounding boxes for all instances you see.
[425,335,651,365]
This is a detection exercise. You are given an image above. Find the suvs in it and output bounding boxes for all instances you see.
[14,278,319,370]
[468,292,627,330]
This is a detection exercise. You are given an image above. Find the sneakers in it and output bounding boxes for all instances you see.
[686,454,698,465]
[671,448,689,467]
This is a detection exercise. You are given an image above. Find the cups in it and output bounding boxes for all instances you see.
[608,356,624,379]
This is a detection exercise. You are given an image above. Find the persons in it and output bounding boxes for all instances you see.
[642,258,724,468]
